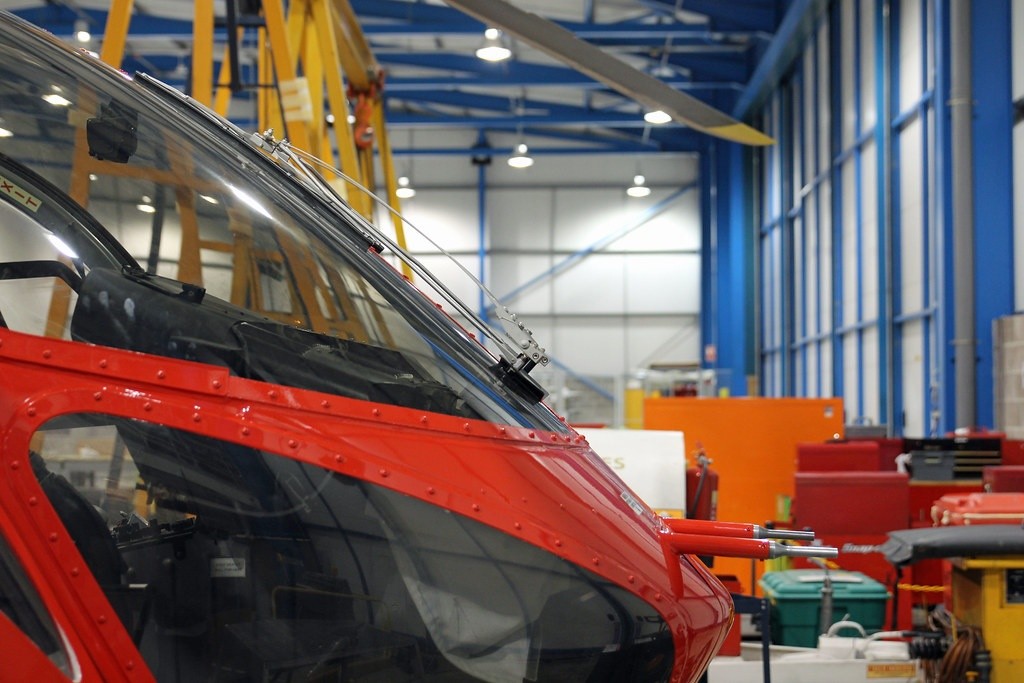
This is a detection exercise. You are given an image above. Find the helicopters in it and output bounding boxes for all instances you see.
[1,0,838,683]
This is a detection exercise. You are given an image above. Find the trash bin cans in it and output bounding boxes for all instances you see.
[759,569,892,650]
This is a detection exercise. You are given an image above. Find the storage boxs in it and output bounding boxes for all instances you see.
[757,426,1024,649]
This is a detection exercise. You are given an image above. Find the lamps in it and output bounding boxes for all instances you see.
[644,103,673,123]
[474,25,511,62]
[507,129,535,167]
[625,151,653,197]
[395,161,416,199]
